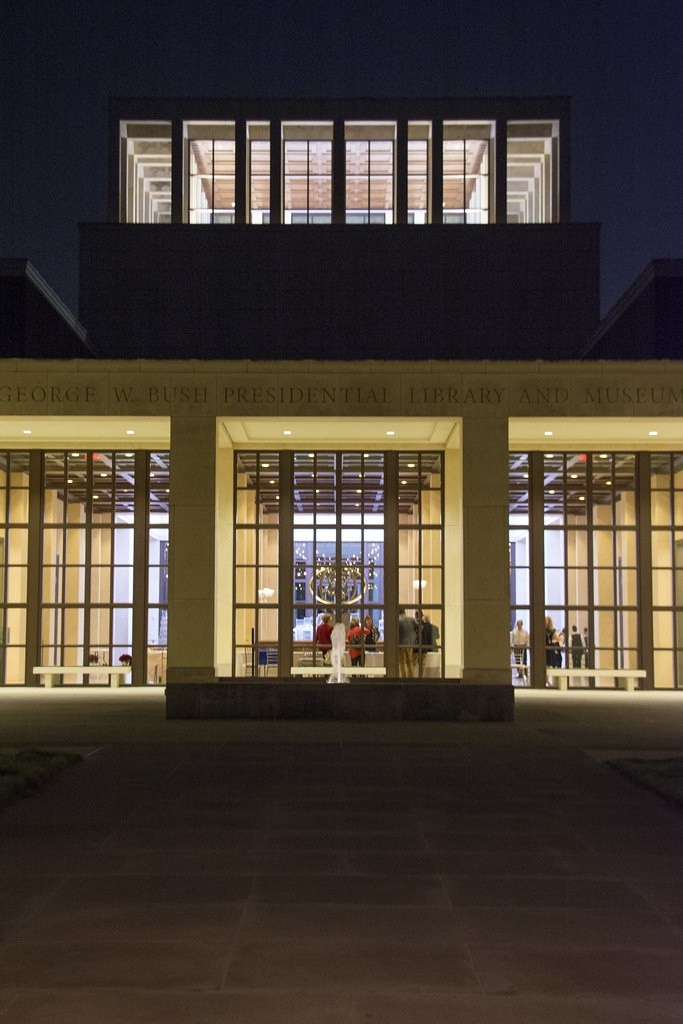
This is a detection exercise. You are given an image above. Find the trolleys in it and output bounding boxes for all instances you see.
[510,644,525,684]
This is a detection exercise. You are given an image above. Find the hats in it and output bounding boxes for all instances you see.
[322,614,332,621]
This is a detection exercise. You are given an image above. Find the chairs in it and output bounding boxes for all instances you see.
[243,647,279,678]
[296,617,313,641]
[301,648,320,678]
[510,649,529,687]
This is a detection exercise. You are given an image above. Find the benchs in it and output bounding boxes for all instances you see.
[547,668,647,692]
[32,666,131,688]
[290,667,387,678]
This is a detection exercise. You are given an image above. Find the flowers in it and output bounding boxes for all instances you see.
[119,654,132,666]
[88,655,98,663]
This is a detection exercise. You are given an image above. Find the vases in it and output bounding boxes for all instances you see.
[89,660,97,666]
[122,660,130,666]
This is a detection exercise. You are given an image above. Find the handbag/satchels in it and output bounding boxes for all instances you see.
[350,634,367,644]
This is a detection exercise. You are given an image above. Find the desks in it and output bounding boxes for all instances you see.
[236,652,255,678]
[147,651,167,685]
[258,651,441,679]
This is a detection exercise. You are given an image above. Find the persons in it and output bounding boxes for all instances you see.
[510,616,592,683]
[308,604,441,682]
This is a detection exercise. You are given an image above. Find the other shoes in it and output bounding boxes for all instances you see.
[516,674,523,679]
